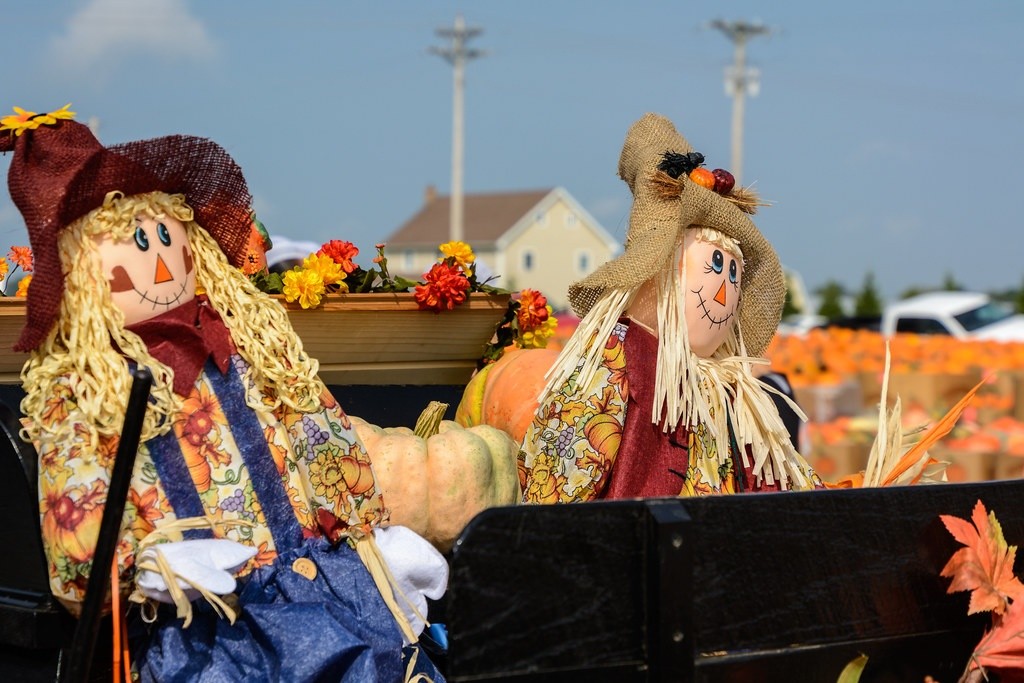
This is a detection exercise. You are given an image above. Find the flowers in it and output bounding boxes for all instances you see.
[0,244,39,300]
[243,236,558,371]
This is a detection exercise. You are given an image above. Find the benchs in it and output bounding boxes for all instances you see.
[417,478,1024,683]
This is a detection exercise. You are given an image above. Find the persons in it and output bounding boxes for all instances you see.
[514,115,815,503]
[0,106,448,683]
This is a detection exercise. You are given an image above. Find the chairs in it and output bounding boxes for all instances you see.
[0,383,147,683]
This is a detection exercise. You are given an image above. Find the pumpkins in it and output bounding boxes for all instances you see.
[455,349,563,446]
[343,399,520,551]
